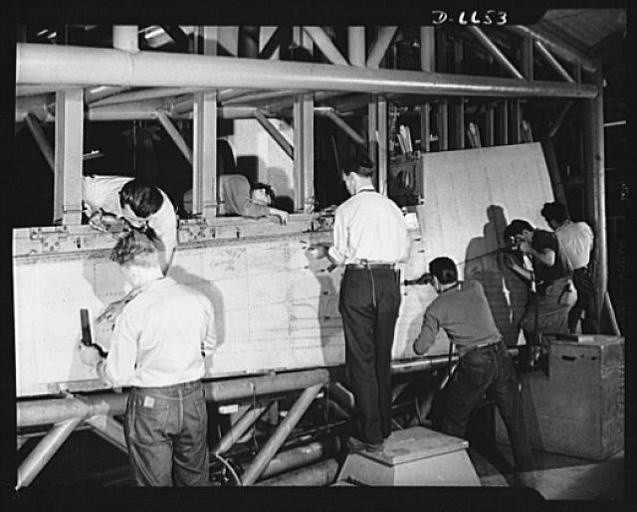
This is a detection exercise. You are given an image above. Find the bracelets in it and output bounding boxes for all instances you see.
[96,361,103,378]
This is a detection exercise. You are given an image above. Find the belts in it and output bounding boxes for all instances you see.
[345,263,396,272]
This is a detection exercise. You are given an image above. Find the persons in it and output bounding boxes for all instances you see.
[81,174,178,325]
[541,201,600,336]
[181,173,289,225]
[413,256,530,473]
[501,220,578,346]
[78,230,218,487]
[308,152,411,453]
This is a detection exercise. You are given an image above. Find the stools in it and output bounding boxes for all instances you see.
[337,426,483,487]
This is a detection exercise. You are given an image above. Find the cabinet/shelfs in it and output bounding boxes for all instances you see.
[494,334,629,462]
